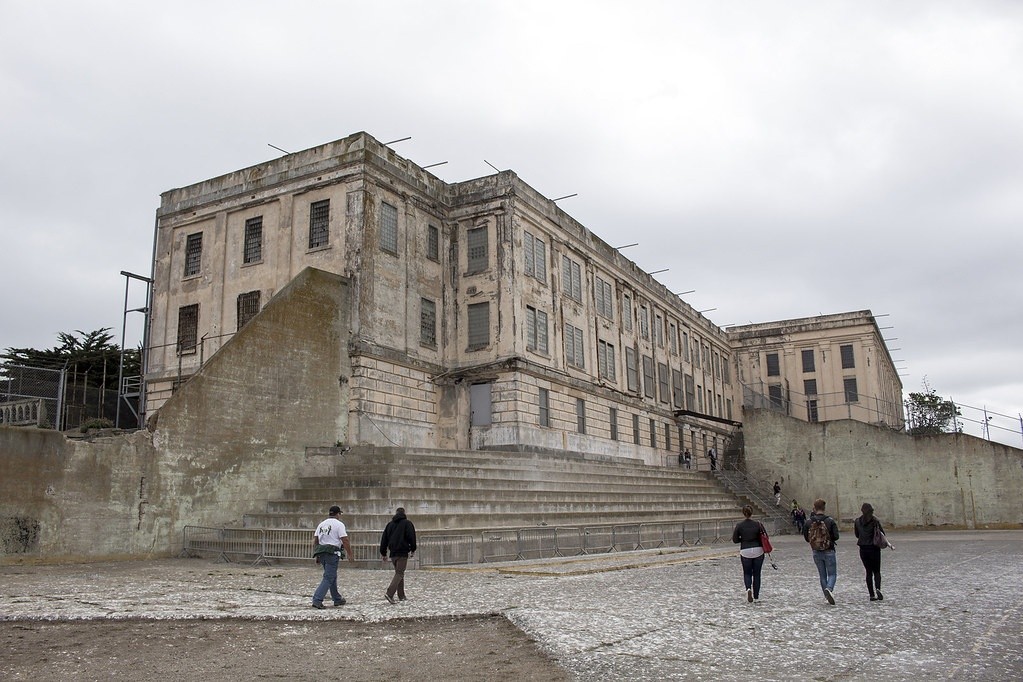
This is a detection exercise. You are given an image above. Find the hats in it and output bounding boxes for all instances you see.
[330,505,344,514]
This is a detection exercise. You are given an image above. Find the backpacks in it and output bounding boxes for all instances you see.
[797,509,804,518]
[809,516,832,551]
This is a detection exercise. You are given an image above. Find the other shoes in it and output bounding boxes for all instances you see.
[870,597,878,601]
[876,588,883,600]
[746,588,753,603]
[825,588,836,605]
[312,602,326,609]
[401,597,407,600]
[385,594,398,605]
[334,598,346,606]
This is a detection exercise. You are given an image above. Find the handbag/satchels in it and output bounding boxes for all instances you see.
[874,517,887,549]
[759,521,773,553]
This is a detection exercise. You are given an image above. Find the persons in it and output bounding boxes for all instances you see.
[733,505,765,602]
[803,498,840,605]
[380,507,417,603]
[311,506,351,610]
[711,445,716,470]
[791,499,806,535]
[854,502,884,601]
[679,448,691,469]
[773,482,781,507]
